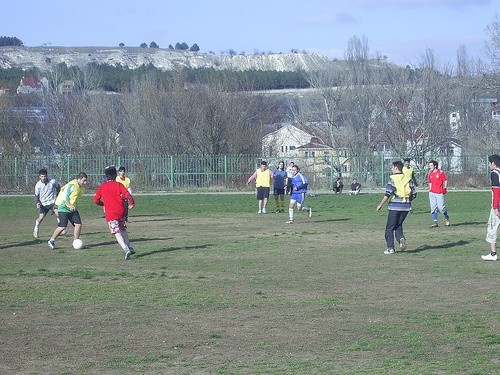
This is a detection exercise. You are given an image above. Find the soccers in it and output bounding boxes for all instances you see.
[72,239,84,249]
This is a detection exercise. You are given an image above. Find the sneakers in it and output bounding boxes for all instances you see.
[308,207,311,217]
[384,248,395,253]
[286,219,294,224]
[481,253,497,261]
[399,238,406,251]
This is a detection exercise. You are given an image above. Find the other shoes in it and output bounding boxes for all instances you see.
[276,210,281,213]
[258,210,262,214]
[431,224,438,227]
[33,232,39,238]
[125,251,131,260]
[446,216,450,226]
[262,210,266,213]
[129,247,135,254]
[48,240,55,250]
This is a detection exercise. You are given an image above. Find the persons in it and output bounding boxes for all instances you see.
[115,167,132,223]
[101,166,110,218]
[246,161,275,214]
[348,178,361,197]
[332,178,343,194]
[93,166,135,260]
[285,165,312,224]
[33,169,66,238]
[402,158,419,214]
[426,160,450,227]
[285,162,294,196]
[481,154,500,261]
[376,160,417,254]
[271,161,287,213]
[47,172,87,250]
